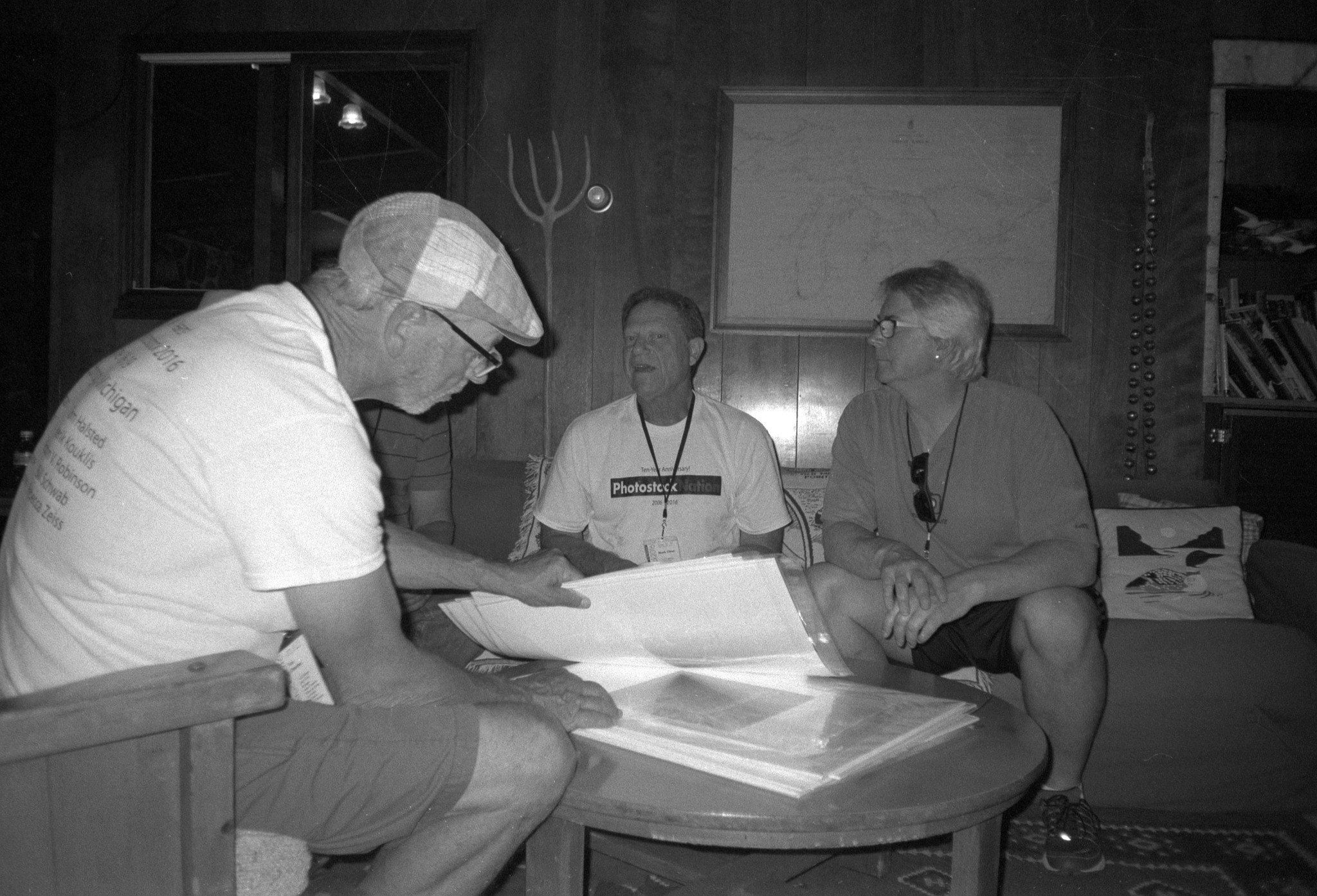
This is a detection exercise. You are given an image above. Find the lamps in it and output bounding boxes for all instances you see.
[338,103,367,130]
[311,76,332,106]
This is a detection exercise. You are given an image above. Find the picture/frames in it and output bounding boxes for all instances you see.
[708,85,1082,344]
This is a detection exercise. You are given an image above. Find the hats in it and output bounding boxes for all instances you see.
[338,193,544,347]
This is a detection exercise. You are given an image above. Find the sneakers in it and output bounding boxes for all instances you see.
[1039,799,1107,873]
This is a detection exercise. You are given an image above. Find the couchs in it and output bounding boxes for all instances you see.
[400,456,1316,819]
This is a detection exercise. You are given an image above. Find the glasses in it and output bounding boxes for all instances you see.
[910,452,936,523]
[871,318,927,338]
[420,306,504,378]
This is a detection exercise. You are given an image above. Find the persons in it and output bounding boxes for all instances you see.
[0,187,623,896]
[802,256,1111,872]
[530,282,798,583]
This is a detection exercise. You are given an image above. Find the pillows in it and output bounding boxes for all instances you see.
[1115,491,1262,612]
[506,456,592,567]
[1094,505,1256,622]
[772,464,830,571]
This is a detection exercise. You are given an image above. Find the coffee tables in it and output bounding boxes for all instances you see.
[486,654,1048,896]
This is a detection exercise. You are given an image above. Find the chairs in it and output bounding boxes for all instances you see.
[0,648,312,896]
